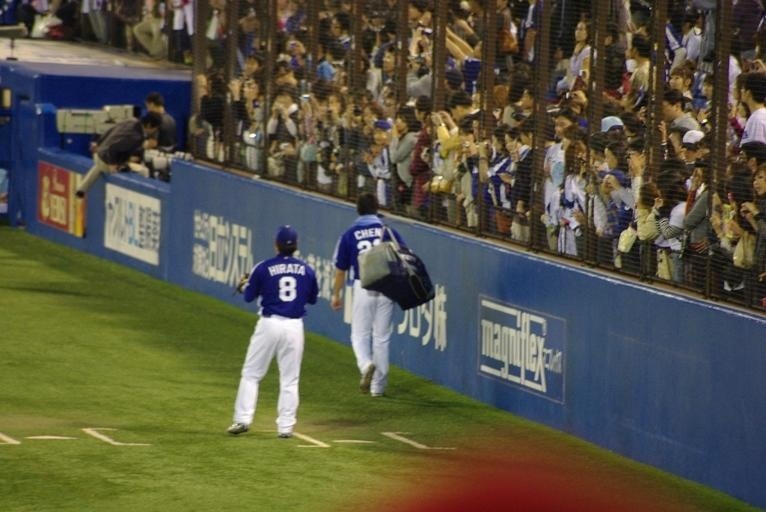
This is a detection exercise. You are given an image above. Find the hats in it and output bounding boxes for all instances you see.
[277,225,297,241]
[601,115,624,133]
[682,130,707,149]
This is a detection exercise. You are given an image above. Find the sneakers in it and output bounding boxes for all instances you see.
[360,364,375,392]
[228,423,247,433]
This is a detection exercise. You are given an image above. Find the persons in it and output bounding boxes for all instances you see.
[227,224,319,438]
[331,193,407,397]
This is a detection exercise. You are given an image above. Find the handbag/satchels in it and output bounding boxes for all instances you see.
[357,242,435,310]
